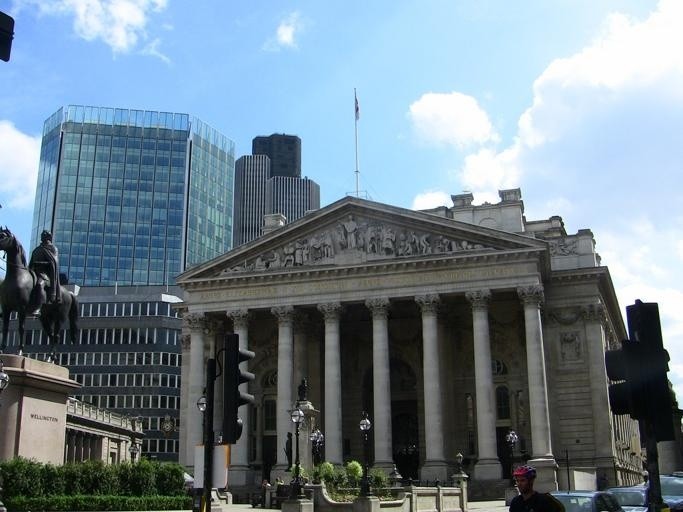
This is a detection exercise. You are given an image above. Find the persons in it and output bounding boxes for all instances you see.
[260,478,271,508]
[285,432,292,468]
[256,214,450,275]
[26,229,59,316]
[509,464,566,512]
[641,468,649,486]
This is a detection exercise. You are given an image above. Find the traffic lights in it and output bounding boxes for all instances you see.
[605,339,639,420]
[222,333,256,445]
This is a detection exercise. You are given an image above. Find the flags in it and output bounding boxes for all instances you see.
[355,97,363,122]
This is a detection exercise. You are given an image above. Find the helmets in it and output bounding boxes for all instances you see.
[514,465,538,480]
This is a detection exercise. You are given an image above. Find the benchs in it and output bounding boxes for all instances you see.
[252,487,266,508]
[270,484,300,509]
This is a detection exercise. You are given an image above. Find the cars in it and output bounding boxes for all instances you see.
[547,472,683,512]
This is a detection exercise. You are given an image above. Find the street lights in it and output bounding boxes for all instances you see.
[506,430,519,487]
[454,453,465,474]
[129,441,138,466]
[309,423,324,477]
[291,403,306,499]
[357,409,374,496]
